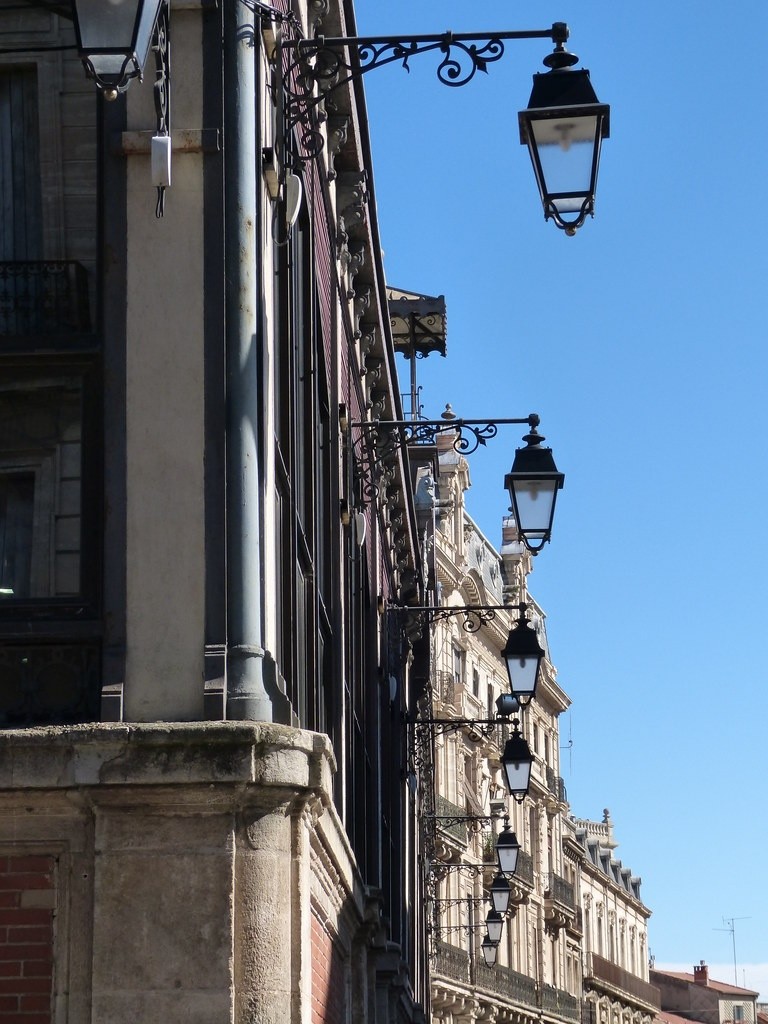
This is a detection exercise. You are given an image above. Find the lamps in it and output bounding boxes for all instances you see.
[380,601,544,711]
[420,812,519,881]
[404,715,536,804]
[340,412,565,557]
[496,693,519,715]
[430,861,514,914]
[270,23,613,237]
[429,895,505,948]
[73,0,173,216]
[430,924,499,970]
